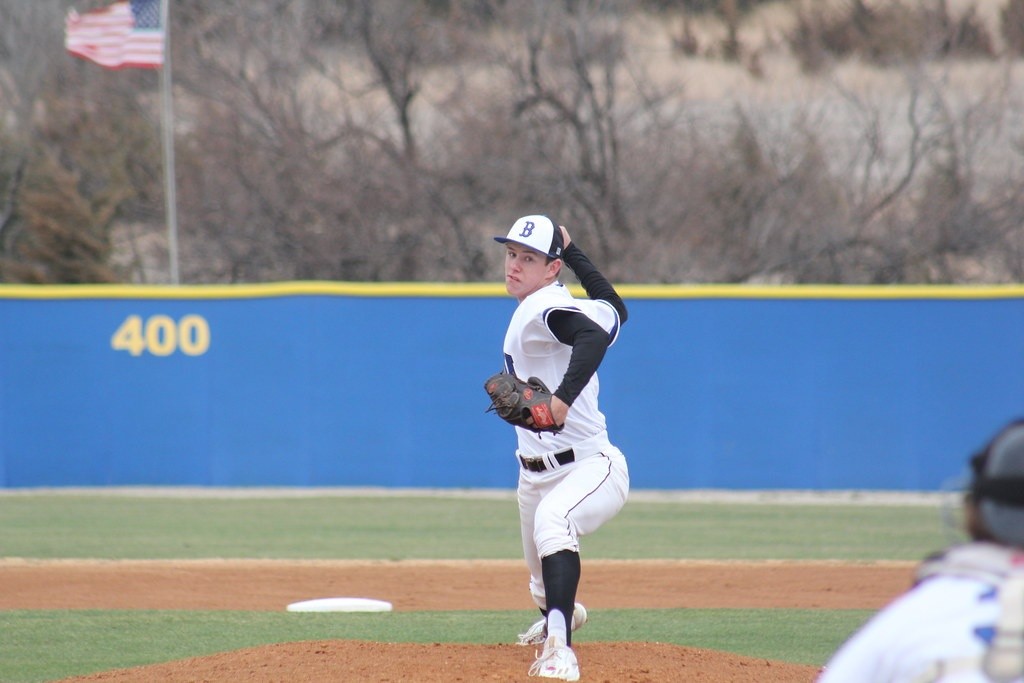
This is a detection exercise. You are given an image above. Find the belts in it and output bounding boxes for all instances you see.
[519,448,574,472]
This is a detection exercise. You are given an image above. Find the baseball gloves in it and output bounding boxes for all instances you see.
[485,374,565,433]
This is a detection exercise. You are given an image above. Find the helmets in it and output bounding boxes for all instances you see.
[961,420,1024,549]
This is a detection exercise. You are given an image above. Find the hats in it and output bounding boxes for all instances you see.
[493,213,563,259]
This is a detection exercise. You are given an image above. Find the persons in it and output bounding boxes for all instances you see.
[483,215,629,681]
[818,415,1024,683]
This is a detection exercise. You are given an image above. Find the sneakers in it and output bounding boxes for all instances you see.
[528,635,580,681]
[514,602,587,646]
